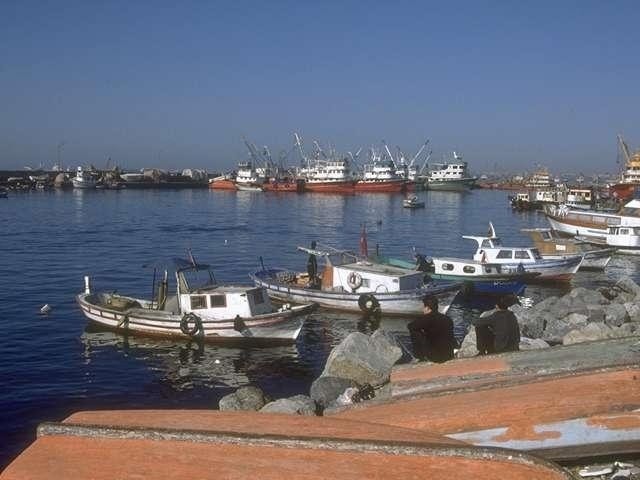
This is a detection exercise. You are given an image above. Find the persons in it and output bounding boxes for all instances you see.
[472,296,520,355]
[407,295,454,362]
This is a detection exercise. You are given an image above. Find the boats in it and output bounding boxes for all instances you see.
[262,169,306,193]
[424,151,478,191]
[249,232,465,316]
[509,133,640,268]
[235,160,266,192]
[301,139,356,194]
[353,147,412,194]
[75,247,320,349]
[386,218,584,296]
[382,140,433,191]
[402,196,426,209]
[0,157,237,199]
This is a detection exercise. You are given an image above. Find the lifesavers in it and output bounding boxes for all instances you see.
[347,272,362,289]
